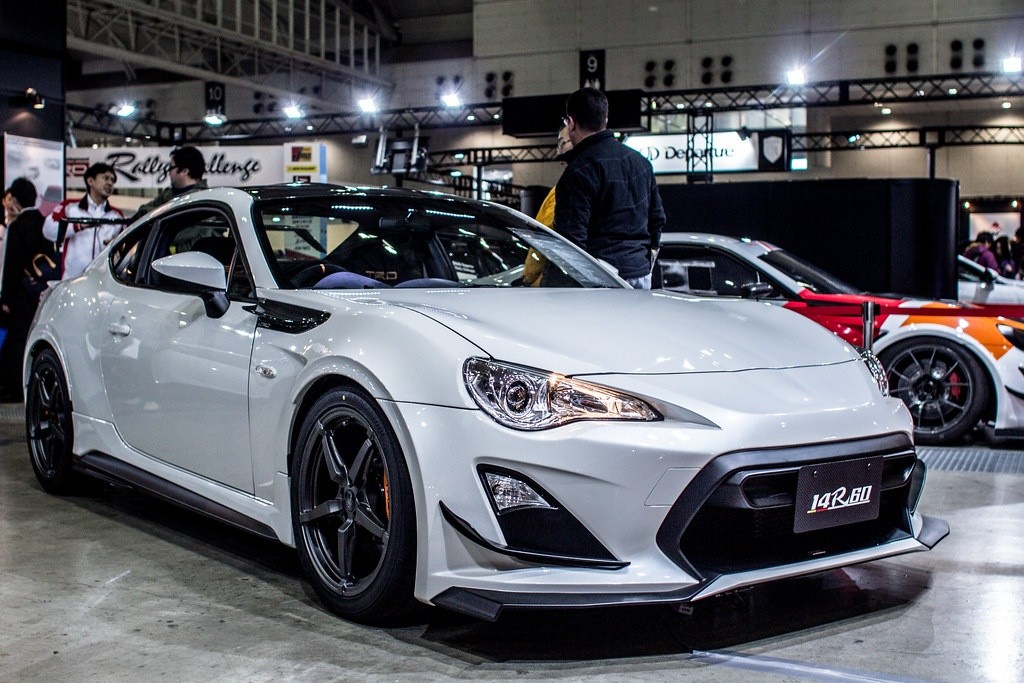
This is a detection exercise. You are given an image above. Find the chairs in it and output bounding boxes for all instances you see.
[189,236,242,265]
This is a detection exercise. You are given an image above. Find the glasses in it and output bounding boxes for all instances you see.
[168,165,177,172]
[559,139,571,149]
[561,117,570,126]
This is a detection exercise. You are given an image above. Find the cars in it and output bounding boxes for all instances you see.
[957,253,1024,306]
[461,232,1024,444]
[23,184,951,621]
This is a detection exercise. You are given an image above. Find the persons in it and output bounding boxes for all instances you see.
[0,175,54,405]
[963,219,1024,280]
[40,161,125,285]
[522,85,668,290]
[103,144,228,254]
